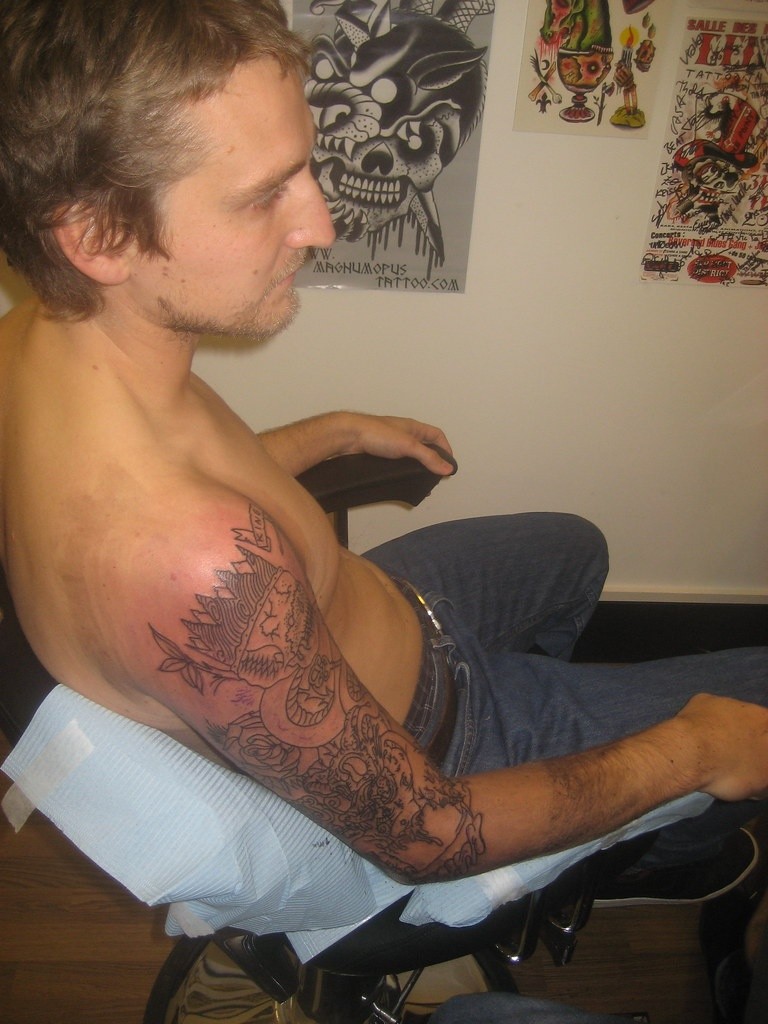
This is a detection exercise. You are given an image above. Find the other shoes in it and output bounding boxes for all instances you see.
[592,828,760,908]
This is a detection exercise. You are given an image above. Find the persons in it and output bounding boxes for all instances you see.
[0,0,768,1024]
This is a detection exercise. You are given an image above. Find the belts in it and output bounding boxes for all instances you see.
[375,564,461,768]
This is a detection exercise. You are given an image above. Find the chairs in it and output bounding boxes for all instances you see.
[0,444,768,1024]
